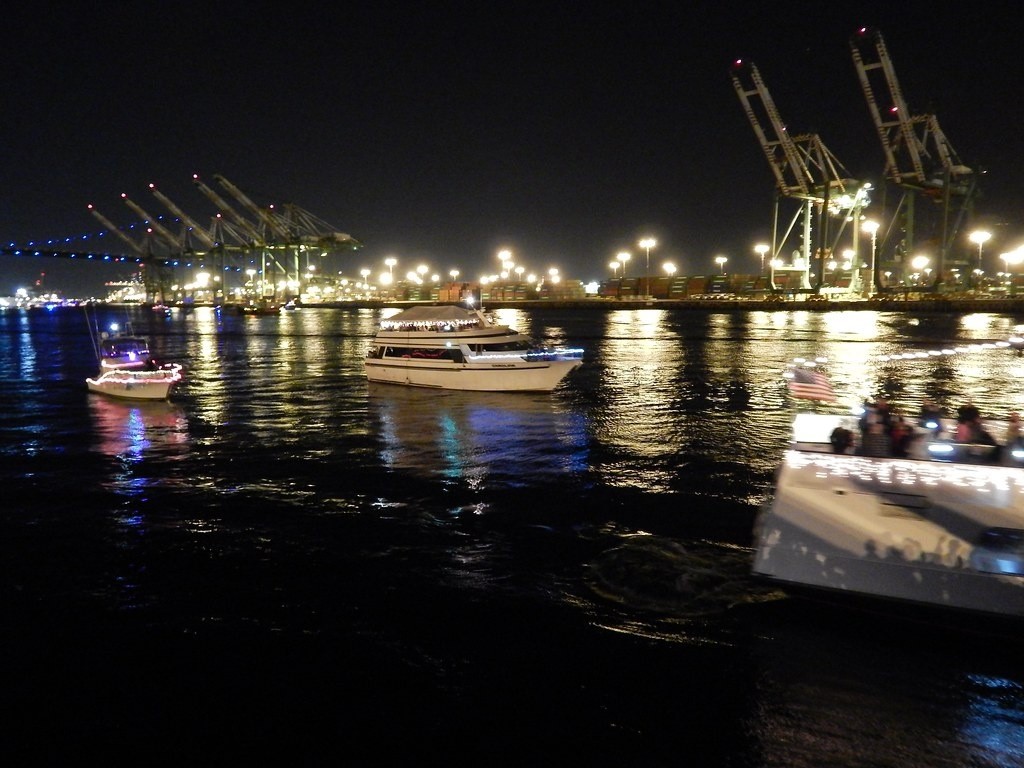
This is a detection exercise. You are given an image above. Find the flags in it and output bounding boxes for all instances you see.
[791,371,837,405]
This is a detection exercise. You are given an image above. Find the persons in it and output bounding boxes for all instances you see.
[828,426,858,457]
[857,411,902,459]
[918,396,942,440]
[860,394,912,455]
[956,405,996,451]
[1003,410,1024,447]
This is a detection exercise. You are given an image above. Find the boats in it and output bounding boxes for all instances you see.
[750,414,1024,616]
[364,306,584,395]
[84,331,183,403]
